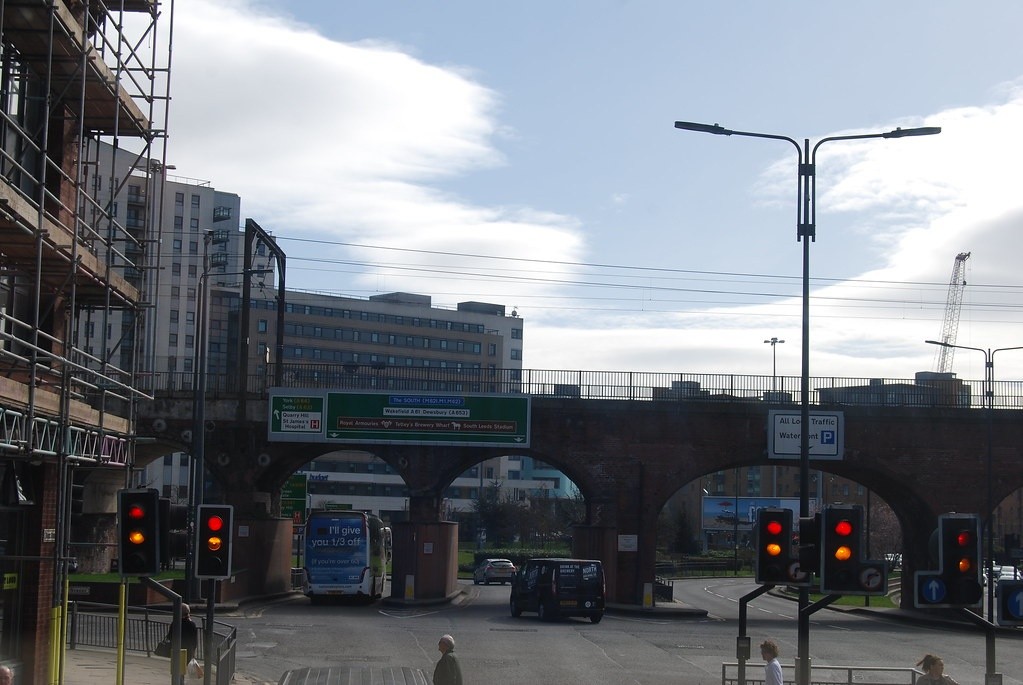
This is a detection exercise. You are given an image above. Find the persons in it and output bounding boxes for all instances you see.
[760,639,783,685]
[433,634,464,685]
[916,653,960,685]
[0,662,16,685]
[165,603,198,685]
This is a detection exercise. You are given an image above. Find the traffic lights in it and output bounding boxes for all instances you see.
[117,489,160,576]
[194,505,232,579]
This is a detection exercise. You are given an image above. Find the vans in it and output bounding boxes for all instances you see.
[509,558,607,623]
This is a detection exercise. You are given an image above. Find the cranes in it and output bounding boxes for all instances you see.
[936,251,971,373]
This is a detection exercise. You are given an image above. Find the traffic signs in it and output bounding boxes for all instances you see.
[267,386,531,449]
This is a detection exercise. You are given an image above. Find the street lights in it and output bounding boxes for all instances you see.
[756,507,794,583]
[674,119,942,685]
[761,337,787,405]
[404,498,408,511]
[823,505,866,594]
[939,514,983,604]
[188,266,274,603]
[923,340,1023,409]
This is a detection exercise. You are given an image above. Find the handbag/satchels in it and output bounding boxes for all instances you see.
[154,634,174,658]
[187,658,205,682]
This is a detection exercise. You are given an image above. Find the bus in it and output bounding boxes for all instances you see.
[304,509,392,603]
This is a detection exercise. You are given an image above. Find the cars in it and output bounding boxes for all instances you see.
[983,561,1023,595]
[473,558,516,584]
[884,553,902,565]
